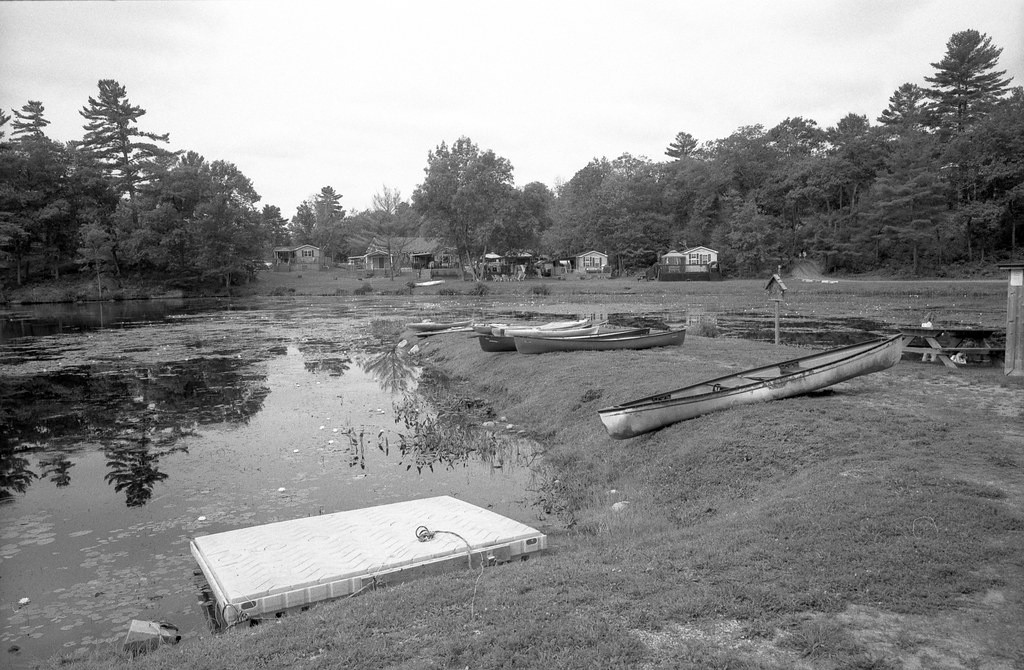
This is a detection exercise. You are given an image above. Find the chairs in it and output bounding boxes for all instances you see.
[491,274,527,282]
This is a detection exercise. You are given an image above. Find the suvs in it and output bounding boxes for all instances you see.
[252,259,273,271]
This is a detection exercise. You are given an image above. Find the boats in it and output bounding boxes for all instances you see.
[472,316,690,354]
[406,280,445,286]
[410,320,473,337]
[598,333,904,439]
